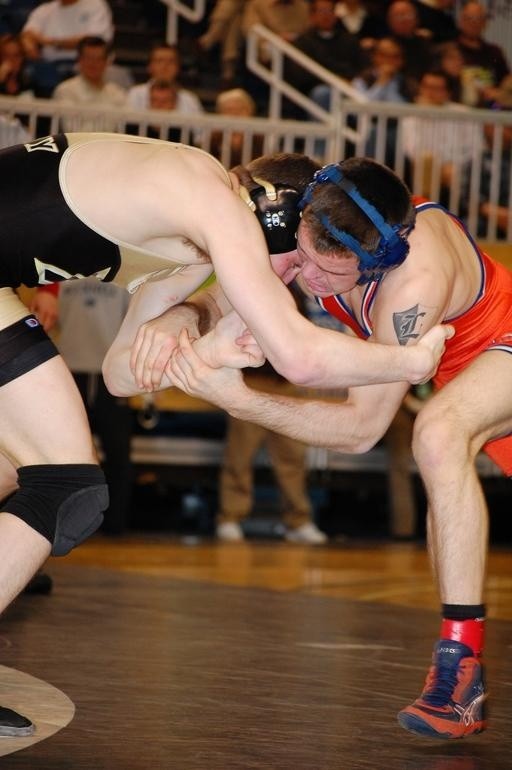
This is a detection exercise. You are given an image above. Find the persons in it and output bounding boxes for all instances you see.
[1,129,458,737]
[130,153,512,738]
[219,280,325,542]
[0,1,511,237]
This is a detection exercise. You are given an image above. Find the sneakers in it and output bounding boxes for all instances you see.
[217,523,240,543]
[0,706,35,736]
[287,523,325,545]
[399,638,487,739]
[22,571,52,596]
